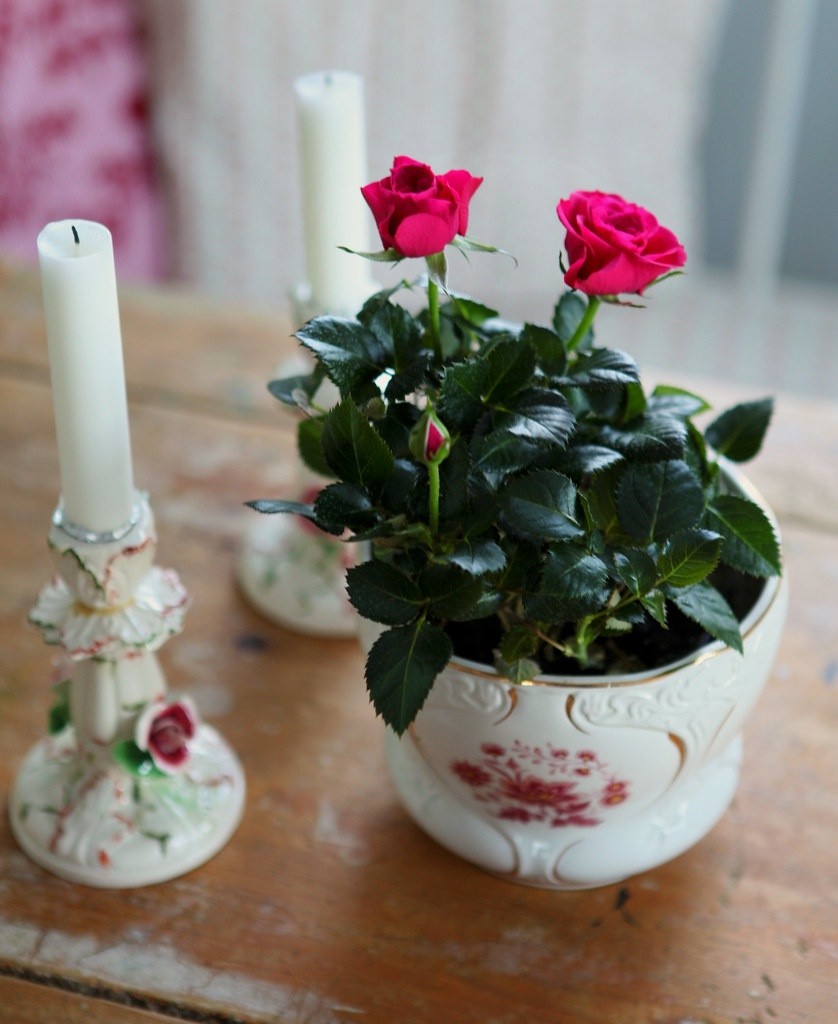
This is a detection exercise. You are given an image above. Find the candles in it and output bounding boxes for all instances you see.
[34,220,136,529]
[290,69,373,316]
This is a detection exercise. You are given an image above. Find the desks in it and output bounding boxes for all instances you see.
[2,271,838,1021]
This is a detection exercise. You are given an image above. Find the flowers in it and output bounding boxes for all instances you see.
[239,152,777,737]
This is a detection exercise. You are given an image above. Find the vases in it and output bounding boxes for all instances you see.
[382,457,791,890]
